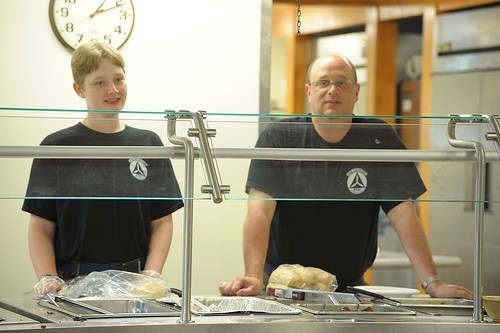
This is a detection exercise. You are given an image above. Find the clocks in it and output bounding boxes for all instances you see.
[48,0,135,51]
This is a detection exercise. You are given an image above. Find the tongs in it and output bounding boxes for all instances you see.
[267,286,374,303]
[45,292,110,314]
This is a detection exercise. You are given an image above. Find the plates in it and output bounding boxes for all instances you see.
[355,285,420,298]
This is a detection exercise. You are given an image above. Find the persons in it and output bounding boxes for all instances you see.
[219,54,475,300]
[22,41,184,297]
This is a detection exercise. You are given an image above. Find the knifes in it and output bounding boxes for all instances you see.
[346,285,401,305]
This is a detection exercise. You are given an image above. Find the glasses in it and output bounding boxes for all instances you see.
[310,79,358,89]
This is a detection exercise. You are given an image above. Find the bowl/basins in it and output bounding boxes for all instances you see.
[483,296,500,323]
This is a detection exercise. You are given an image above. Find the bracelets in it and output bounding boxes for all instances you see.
[422,275,440,288]
